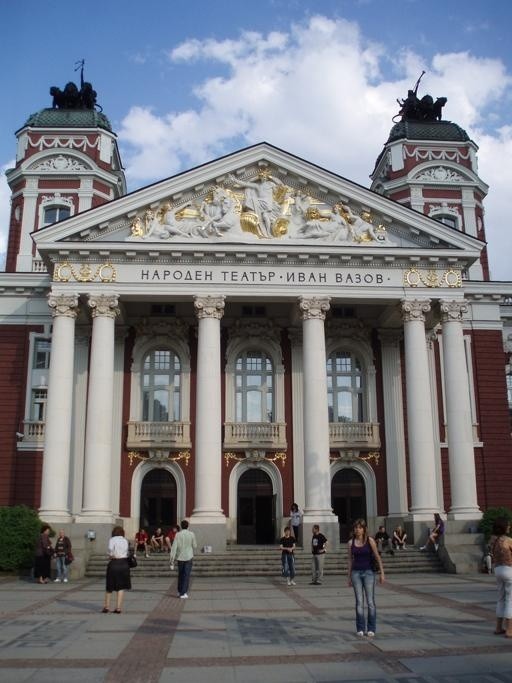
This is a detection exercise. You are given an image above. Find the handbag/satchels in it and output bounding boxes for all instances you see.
[65,553,73,562]
[127,554,137,567]
[368,548,379,571]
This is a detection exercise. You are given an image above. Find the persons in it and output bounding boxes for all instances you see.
[280,504,327,585]
[348,519,384,637]
[34,525,53,584]
[490,517,511,638]
[133,524,180,557]
[170,520,197,598]
[53,529,74,583]
[142,171,384,243]
[375,513,444,555]
[104,526,129,613]
